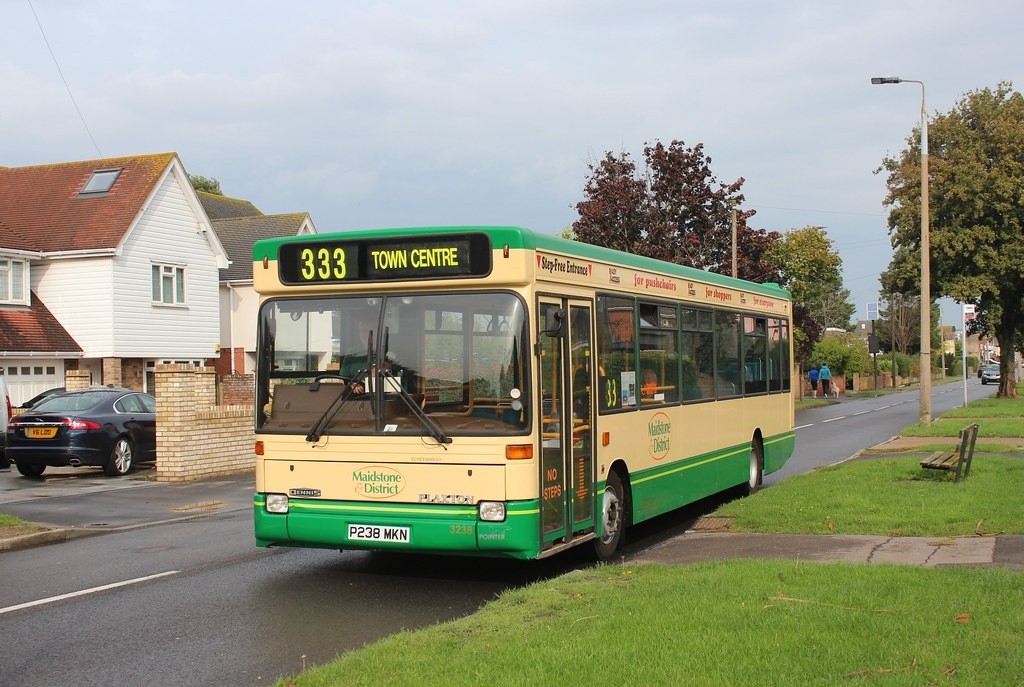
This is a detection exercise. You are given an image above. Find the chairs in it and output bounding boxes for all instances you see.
[729,356,771,382]
[59,396,145,416]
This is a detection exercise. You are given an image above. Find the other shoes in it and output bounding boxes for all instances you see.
[824,393,828,399]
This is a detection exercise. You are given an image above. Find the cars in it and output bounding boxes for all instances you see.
[4,389,156,477]
[977,359,1001,385]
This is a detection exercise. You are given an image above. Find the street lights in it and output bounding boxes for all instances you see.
[871,77,930,426]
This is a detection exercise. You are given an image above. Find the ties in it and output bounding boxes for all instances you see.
[366,347,372,369]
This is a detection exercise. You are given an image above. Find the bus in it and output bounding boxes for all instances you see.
[253,224,793,564]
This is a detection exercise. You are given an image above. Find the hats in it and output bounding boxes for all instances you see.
[357,319,378,332]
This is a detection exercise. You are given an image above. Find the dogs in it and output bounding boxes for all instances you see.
[832,387,840,398]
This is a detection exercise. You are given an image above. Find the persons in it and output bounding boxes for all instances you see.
[336,315,393,395]
[817,363,834,398]
[807,364,819,399]
[640,368,658,398]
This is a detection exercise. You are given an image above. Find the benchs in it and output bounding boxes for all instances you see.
[918,422,982,483]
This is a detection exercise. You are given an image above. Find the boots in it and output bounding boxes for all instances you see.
[812,389,818,398]
